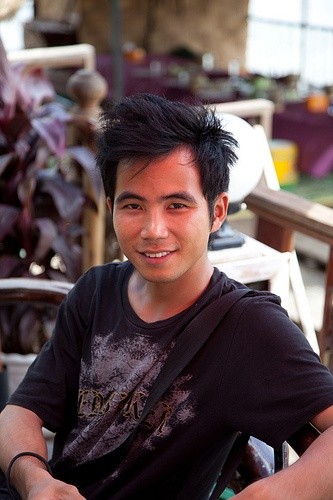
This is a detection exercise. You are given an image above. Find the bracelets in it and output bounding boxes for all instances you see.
[6,452,51,500]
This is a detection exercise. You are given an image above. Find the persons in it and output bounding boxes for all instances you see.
[0,94,333,500]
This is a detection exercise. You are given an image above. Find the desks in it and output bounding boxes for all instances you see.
[95,52,333,179]
[209,232,319,353]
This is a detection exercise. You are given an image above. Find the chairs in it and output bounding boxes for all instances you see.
[0,278,333,500]
